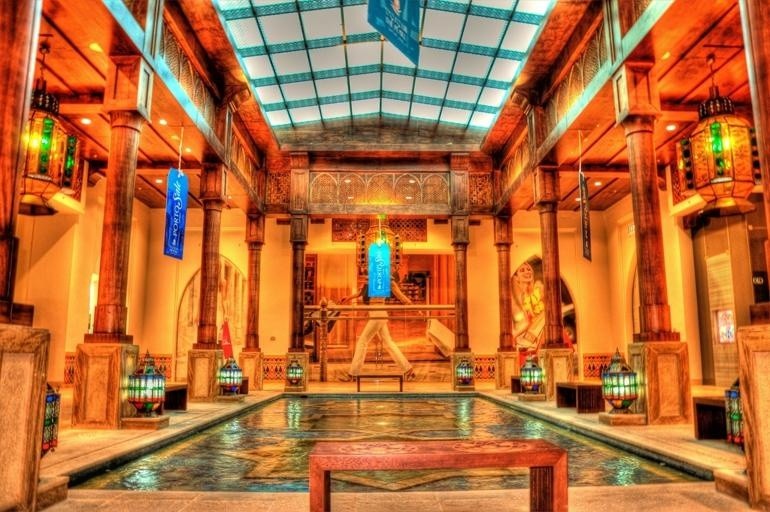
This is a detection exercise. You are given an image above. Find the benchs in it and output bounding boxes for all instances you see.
[308,439,569,511]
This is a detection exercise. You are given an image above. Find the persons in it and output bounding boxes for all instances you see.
[513,261,547,349]
[336,277,426,384]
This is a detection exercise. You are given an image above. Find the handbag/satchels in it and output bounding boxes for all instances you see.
[312,300,340,333]
[427,318,456,357]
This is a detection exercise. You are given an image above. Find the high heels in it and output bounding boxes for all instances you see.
[339,374,358,382]
[404,371,416,381]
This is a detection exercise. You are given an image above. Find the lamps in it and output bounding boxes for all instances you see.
[689,54,756,219]
[20,33,68,207]
[39,348,305,458]
[365,225,397,274]
[454,347,745,451]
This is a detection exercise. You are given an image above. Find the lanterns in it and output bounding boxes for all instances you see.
[688,97,758,221]
[17,89,69,218]
